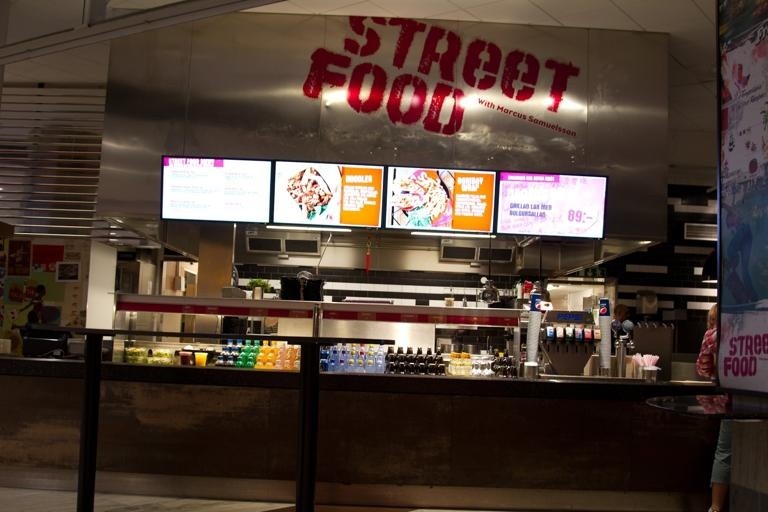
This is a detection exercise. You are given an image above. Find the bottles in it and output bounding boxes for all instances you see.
[516,281,541,299]
[33,293,43,311]
[327,338,445,375]
[448,352,473,376]
[492,348,518,378]
[214,339,301,370]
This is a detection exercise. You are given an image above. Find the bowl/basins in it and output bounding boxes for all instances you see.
[464,344,479,355]
[441,343,458,354]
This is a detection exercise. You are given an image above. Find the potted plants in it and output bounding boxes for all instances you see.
[248,279,270,299]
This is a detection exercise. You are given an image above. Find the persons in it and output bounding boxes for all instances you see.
[696,302,732,512]
[17,284,46,325]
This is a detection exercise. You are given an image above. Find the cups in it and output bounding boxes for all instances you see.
[526,293,544,361]
[40,264,46,272]
[640,367,657,382]
[179,352,193,366]
[445,298,455,307]
[472,354,495,378]
[599,298,612,368]
[252,287,264,301]
[195,352,208,367]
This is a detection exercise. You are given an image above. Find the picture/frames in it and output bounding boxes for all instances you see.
[55,262,81,282]
